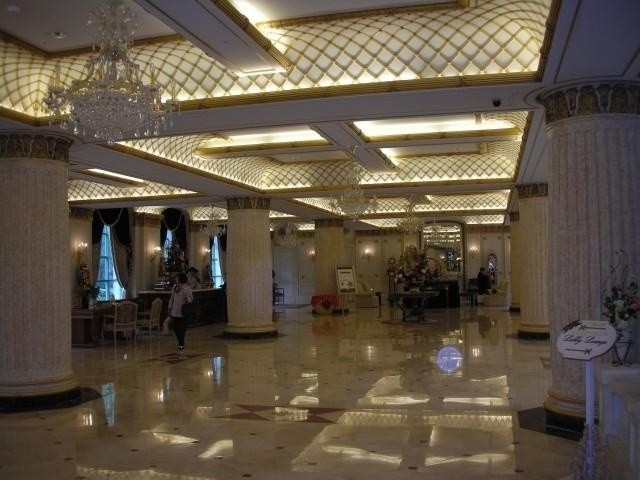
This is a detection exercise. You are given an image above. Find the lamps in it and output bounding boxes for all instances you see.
[37,0,183,149]
[328,144,377,220]
[199,202,227,238]
[423,213,445,247]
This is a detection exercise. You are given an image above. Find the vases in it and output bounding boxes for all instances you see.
[609,300,633,366]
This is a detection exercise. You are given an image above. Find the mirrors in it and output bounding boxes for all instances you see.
[422,223,462,273]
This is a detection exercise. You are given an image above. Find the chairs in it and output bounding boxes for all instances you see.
[137,297,163,341]
[99,301,139,348]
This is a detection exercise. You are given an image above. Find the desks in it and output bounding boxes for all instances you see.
[596,364,640,472]
[392,291,439,324]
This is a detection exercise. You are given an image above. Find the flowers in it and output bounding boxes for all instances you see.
[597,248,640,340]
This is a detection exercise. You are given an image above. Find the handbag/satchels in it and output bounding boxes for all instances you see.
[181,299,192,316]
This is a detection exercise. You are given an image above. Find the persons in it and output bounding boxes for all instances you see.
[477,268,485,294]
[186,267,200,290]
[168,273,193,349]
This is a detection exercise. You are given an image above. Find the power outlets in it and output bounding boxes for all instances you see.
[394,194,423,234]
